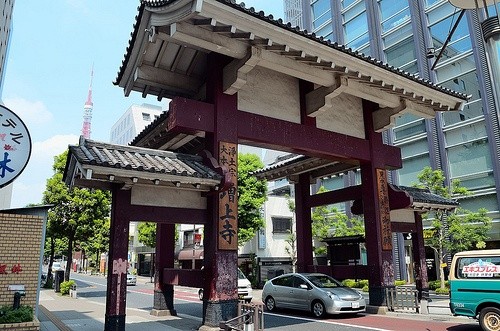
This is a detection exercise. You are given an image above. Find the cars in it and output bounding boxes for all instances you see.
[261,271,367,319]
[53,262,61,270]
[126,272,137,286]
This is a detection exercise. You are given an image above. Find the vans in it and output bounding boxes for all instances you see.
[445,249,500,331]
[198,264,253,303]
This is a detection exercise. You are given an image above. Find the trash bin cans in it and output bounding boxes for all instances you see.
[55,270,64,292]
